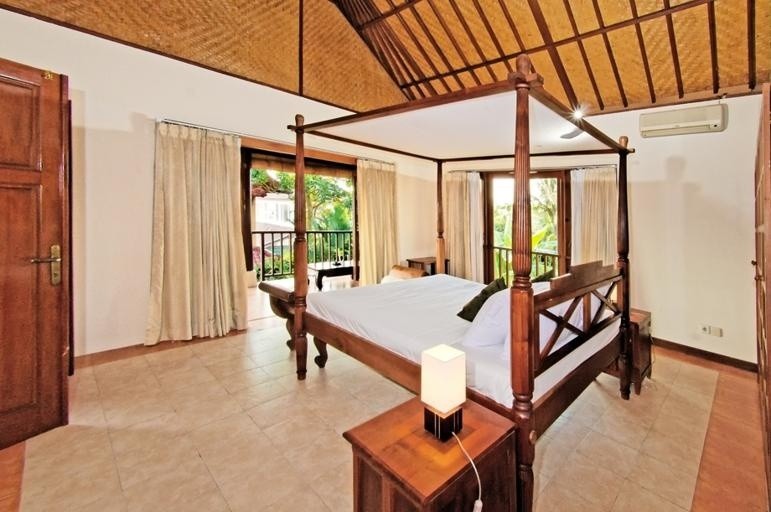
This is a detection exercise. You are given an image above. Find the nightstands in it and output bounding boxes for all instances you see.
[601,306,654,396]
[340,389,517,510]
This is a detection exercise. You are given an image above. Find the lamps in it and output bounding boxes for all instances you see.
[419,343,468,440]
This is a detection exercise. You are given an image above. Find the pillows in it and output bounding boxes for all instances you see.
[455,266,556,362]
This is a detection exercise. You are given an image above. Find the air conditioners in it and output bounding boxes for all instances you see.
[637,104,729,139]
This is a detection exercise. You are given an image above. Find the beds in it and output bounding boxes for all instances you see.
[285,52,636,512]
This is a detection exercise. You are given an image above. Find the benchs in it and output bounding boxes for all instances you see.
[260,264,430,352]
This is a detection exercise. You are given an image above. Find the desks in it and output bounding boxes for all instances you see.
[305,259,354,293]
[406,255,451,276]
